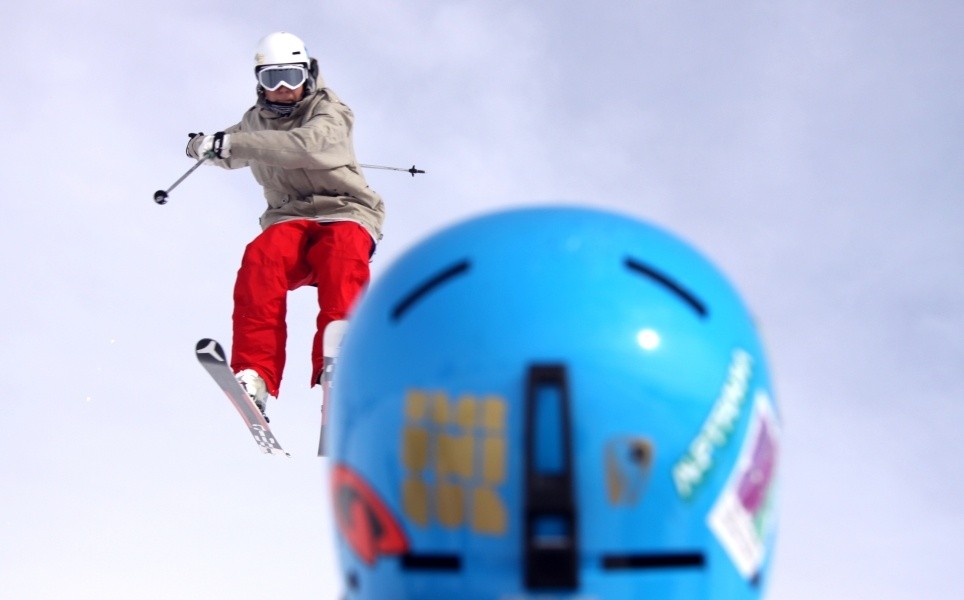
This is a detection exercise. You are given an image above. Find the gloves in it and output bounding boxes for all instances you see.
[197,131,232,162]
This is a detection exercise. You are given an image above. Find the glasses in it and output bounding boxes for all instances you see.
[258,66,307,92]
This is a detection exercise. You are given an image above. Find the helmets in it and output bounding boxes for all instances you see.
[330,202,782,600]
[254,31,311,77]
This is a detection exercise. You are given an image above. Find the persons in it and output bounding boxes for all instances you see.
[328,204,779,600]
[185,33,385,424]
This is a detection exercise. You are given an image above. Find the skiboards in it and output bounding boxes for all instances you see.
[194,318,350,460]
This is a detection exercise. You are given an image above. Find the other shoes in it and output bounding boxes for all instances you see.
[236,370,268,412]
